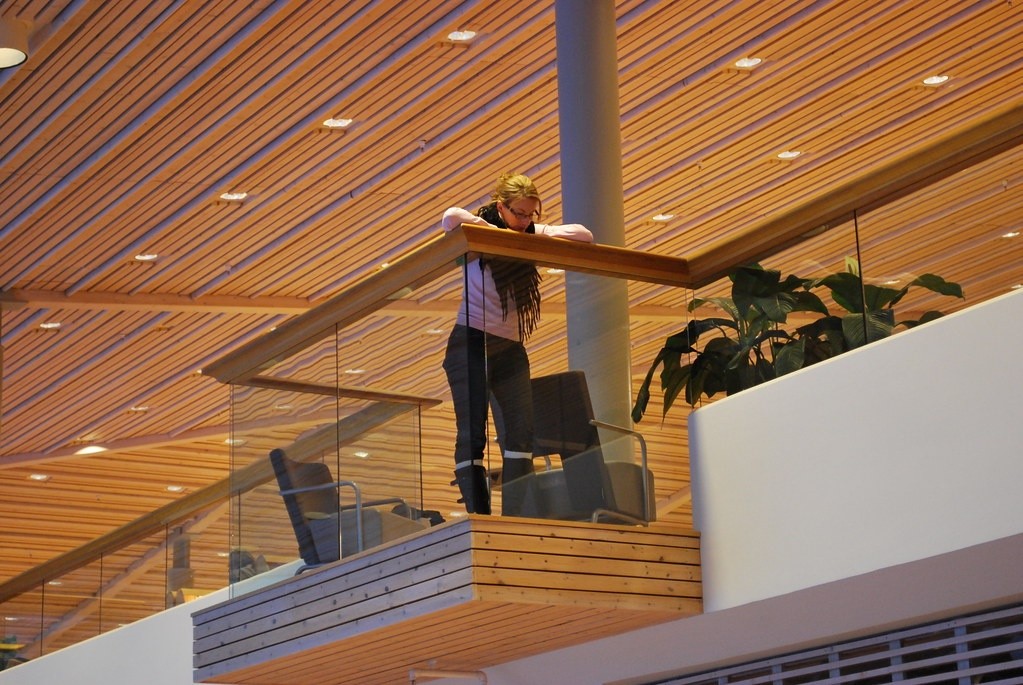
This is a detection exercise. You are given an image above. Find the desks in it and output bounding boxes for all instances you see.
[485,465,562,491]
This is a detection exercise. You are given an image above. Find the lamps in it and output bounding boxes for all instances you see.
[0,18,30,71]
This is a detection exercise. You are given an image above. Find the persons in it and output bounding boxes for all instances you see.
[442,176,594,516]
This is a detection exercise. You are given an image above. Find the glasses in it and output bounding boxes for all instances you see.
[503,202,540,221]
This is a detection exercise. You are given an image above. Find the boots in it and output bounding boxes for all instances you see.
[501,450,533,484]
[450,459,492,515]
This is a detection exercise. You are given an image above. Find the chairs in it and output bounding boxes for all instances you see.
[494,371,656,528]
[269,448,425,577]
